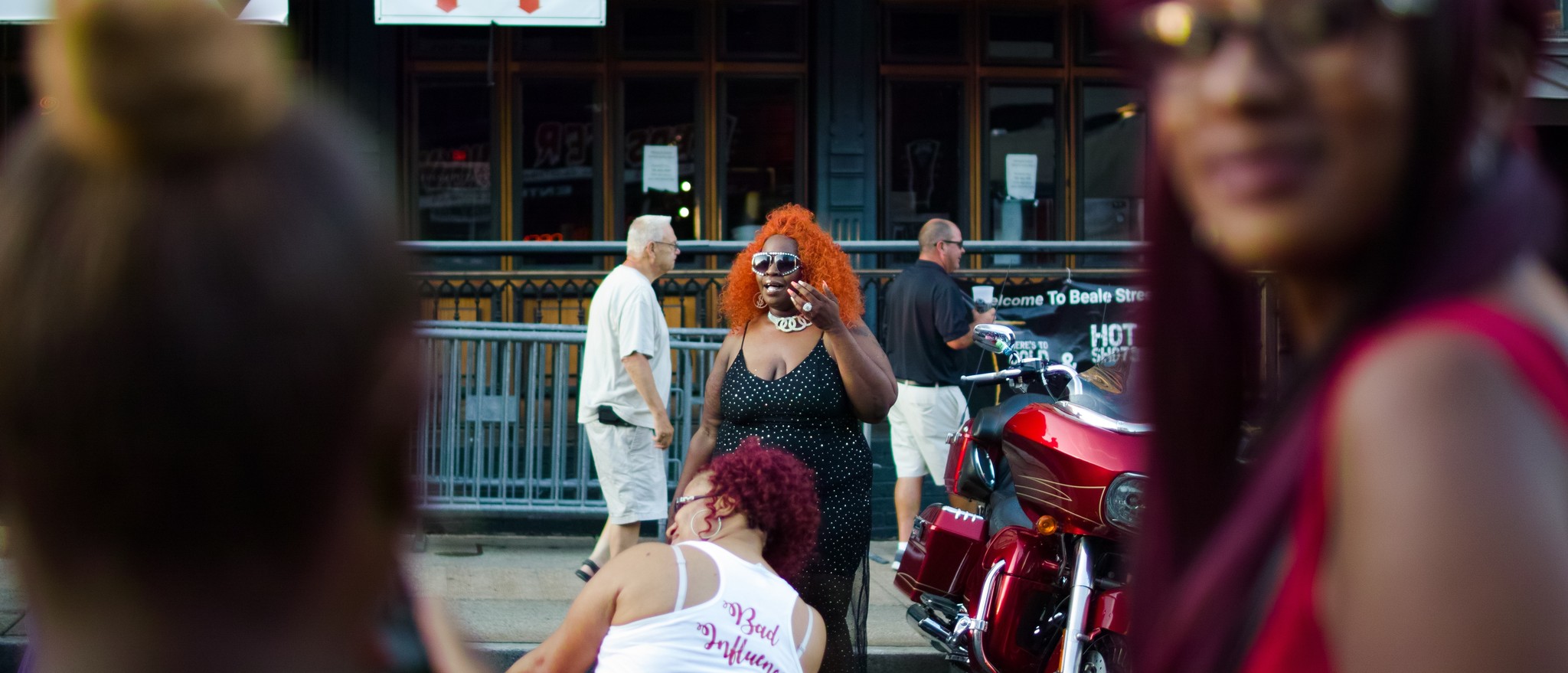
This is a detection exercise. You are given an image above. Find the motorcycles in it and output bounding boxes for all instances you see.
[891,323,1154,673]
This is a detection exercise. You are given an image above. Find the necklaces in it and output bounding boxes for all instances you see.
[767,310,813,332]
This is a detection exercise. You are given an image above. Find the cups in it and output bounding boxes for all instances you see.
[972,285,993,313]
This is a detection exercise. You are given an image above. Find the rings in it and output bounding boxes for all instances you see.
[803,302,812,312]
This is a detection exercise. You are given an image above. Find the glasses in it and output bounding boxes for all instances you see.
[935,240,963,249]
[655,241,679,251]
[674,493,716,512]
[1140,2,1414,46]
[751,252,800,276]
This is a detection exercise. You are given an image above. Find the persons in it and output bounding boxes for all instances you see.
[0,0,436,672]
[1110,0,1568,673]
[505,435,826,673]
[576,215,681,581]
[666,202,898,673]
[880,217,997,569]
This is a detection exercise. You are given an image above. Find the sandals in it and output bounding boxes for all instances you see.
[576,559,600,582]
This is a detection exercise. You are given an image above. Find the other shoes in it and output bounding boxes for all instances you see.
[891,549,904,571]
